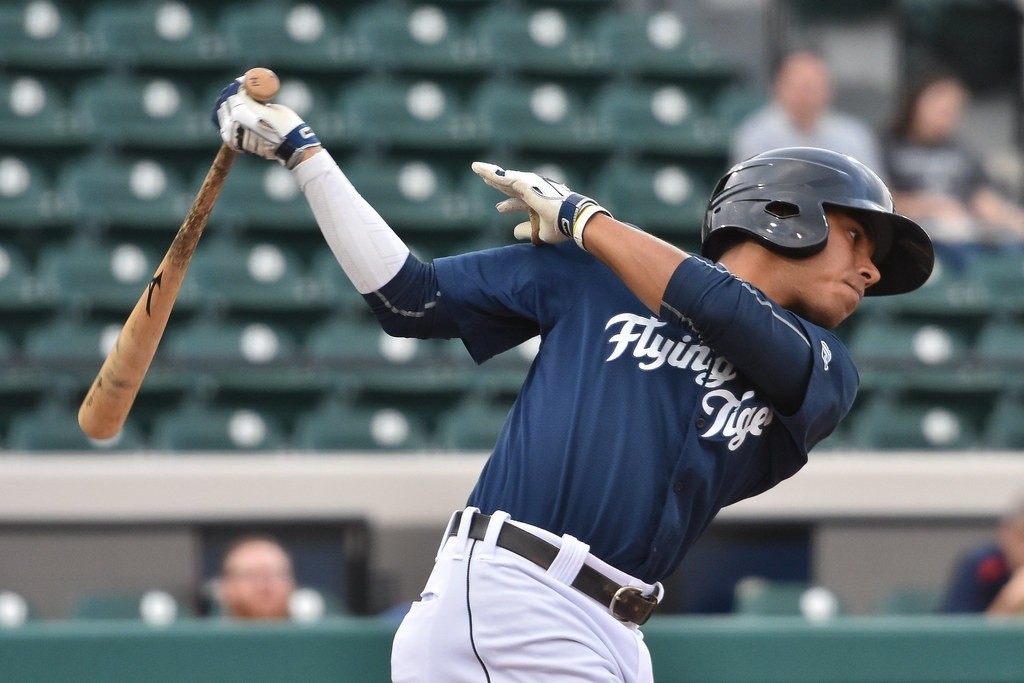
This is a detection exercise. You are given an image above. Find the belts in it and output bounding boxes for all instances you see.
[449,512,658,627]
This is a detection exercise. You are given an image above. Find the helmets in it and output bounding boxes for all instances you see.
[701,147,935,296]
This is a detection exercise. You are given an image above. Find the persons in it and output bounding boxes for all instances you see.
[217,536,296,620]
[207,75,881,683]
[729,51,1023,251]
[927,492,1024,614]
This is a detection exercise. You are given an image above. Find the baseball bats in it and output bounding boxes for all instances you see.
[77,67,279,439]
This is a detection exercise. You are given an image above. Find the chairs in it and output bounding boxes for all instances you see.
[0,586,1024,683]
[0,0,1024,452]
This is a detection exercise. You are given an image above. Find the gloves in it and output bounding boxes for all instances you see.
[211,75,320,169]
[472,161,615,253]
